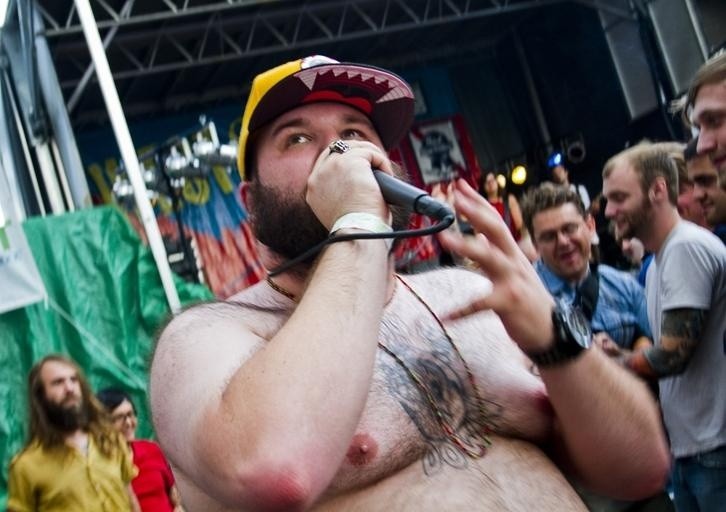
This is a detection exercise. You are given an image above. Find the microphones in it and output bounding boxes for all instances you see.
[372,166,456,223]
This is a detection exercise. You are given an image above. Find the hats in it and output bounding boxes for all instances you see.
[237,54,416,178]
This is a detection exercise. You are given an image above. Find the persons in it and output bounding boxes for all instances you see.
[524,139,725,511]
[680,50,726,190]
[93,385,185,512]
[8,354,141,512]
[150,51,673,512]
[484,164,599,263]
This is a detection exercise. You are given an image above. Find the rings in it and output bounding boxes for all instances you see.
[324,138,353,153]
